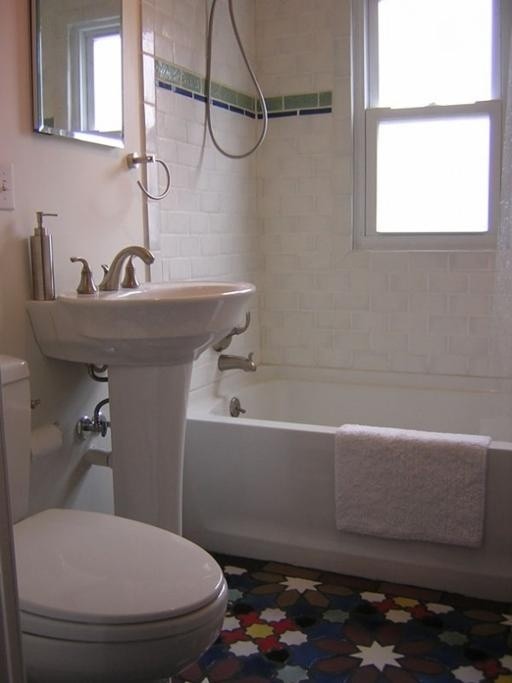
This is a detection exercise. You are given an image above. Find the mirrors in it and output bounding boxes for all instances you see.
[32,2,124,151]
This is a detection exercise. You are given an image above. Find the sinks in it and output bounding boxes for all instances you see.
[24,279,258,370]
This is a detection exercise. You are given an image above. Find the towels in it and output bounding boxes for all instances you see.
[331,420,491,548]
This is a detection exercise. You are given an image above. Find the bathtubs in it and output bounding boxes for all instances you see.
[183,364,511,607]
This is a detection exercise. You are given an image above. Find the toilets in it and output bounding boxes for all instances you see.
[1,354,231,683]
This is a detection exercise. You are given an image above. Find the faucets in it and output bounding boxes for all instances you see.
[97,245,155,291]
